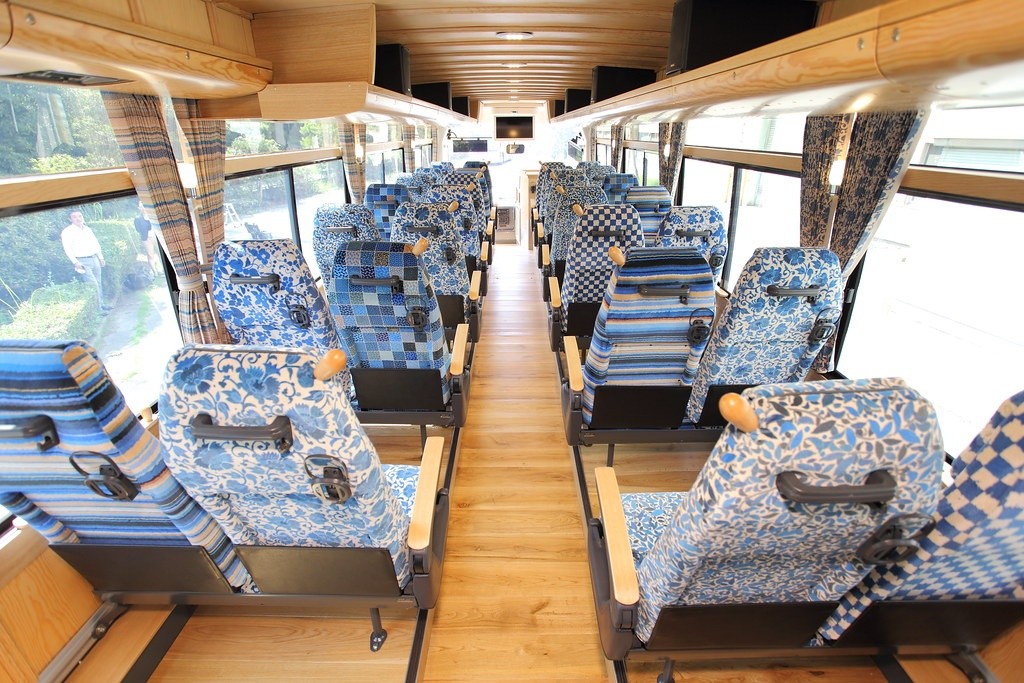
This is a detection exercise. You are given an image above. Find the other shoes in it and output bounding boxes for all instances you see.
[100,304,115,310]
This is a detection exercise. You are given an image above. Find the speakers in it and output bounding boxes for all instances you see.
[374,43,470,116]
[554,0,822,116]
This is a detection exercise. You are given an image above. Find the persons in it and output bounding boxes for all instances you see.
[135,202,164,276]
[61,209,113,315]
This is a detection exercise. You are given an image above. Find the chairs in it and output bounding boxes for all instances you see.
[0,162,495,652]
[538,159,1024,683]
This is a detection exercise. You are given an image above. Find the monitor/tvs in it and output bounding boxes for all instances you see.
[494,115,535,141]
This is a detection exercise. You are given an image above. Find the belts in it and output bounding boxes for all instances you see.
[87,254,96,258]
